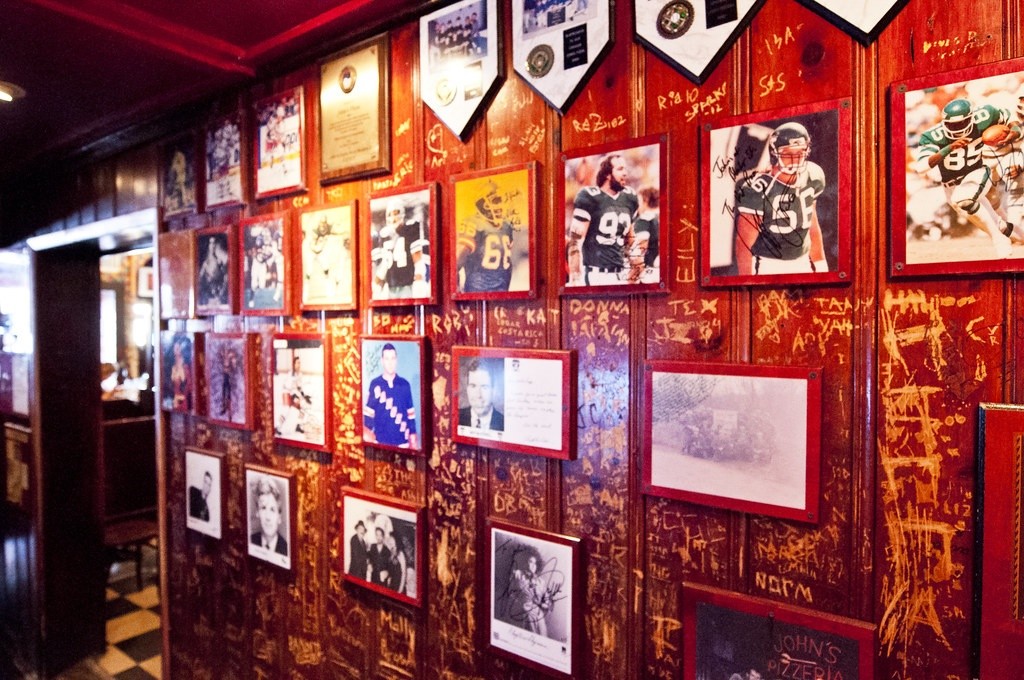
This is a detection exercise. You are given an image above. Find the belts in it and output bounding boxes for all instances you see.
[943,174,965,187]
[586,267,623,273]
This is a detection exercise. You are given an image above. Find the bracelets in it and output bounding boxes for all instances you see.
[938,145,952,158]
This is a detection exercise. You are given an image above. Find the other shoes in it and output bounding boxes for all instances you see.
[275,429,281,435]
[296,426,304,433]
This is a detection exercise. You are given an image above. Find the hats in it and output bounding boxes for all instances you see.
[354,521,367,533]
[514,546,544,577]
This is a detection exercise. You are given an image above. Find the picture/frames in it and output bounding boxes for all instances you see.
[183,446,228,542]
[359,335,433,458]
[160,330,203,418]
[243,463,299,574]
[484,517,585,680]
[681,580,878,680]
[340,485,429,609]
[642,359,824,524]
[160,31,1024,321]
[204,331,262,431]
[452,345,579,462]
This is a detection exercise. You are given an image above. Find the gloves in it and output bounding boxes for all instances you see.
[304,395,312,404]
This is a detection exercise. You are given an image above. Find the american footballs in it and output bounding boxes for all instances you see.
[982,125,1010,146]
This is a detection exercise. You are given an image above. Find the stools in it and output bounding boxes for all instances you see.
[102,521,160,587]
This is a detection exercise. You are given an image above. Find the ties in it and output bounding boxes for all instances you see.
[265,545,269,549]
[476,419,481,429]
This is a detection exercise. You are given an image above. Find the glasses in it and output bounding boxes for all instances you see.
[946,120,968,131]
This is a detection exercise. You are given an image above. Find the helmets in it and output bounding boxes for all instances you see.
[941,99,973,140]
[769,121,810,175]
[384,203,405,227]
[473,183,507,230]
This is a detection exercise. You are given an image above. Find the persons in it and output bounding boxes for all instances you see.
[458,359,504,431]
[346,520,407,598]
[198,233,229,306]
[172,341,191,413]
[376,200,426,298]
[243,233,283,302]
[251,478,288,557]
[189,471,212,523]
[455,184,513,291]
[263,96,295,175]
[207,120,240,199]
[217,340,238,422]
[362,343,418,450]
[735,122,829,276]
[916,87,1024,259]
[275,355,313,434]
[565,153,658,284]
[434,12,481,61]
[534,0,587,28]
[746,667,761,680]
[509,553,549,639]
[170,149,186,210]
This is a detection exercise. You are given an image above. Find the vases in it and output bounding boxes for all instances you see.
[270,334,335,453]
